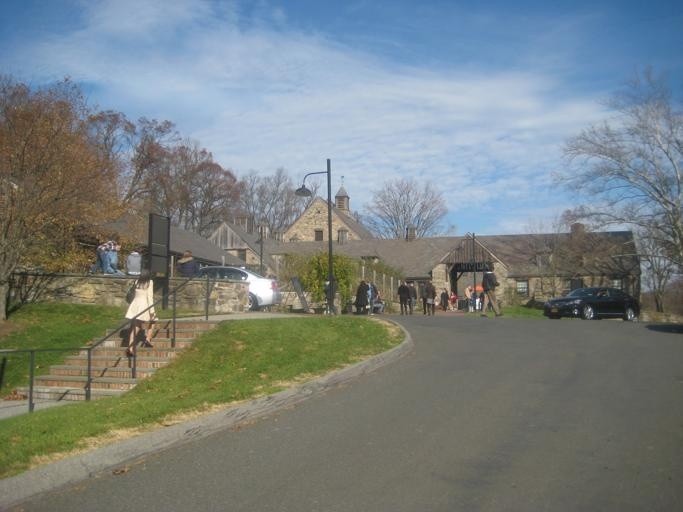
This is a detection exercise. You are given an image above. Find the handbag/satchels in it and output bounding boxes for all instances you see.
[127,280,138,304]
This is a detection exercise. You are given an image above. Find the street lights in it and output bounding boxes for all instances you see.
[456,233,476,311]
[294,159,334,315]
[255,226,263,277]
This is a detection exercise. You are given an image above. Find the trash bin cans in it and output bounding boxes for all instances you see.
[458,300,467,310]
[468,306,473,312]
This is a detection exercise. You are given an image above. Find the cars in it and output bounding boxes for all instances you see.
[542,287,640,321]
[199,265,279,312]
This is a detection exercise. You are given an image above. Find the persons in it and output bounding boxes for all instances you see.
[425,283,436,316]
[450,290,457,308]
[124,268,158,358]
[439,288,448,311]
[353,280,369,313]
[365,280,386,315]
[323,275,338,316]
[396,280,409,314]
[422,281,430,315]
[103,244,124,277]
[405,283,418,314]
[462,284,484,314]
[478,263,503,317]
[86,240,121,277]
[175,249,194,276]
[125,249,142,276]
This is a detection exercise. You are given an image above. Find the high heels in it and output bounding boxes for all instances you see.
[145,340,154,348]
[127,350,138,358]
